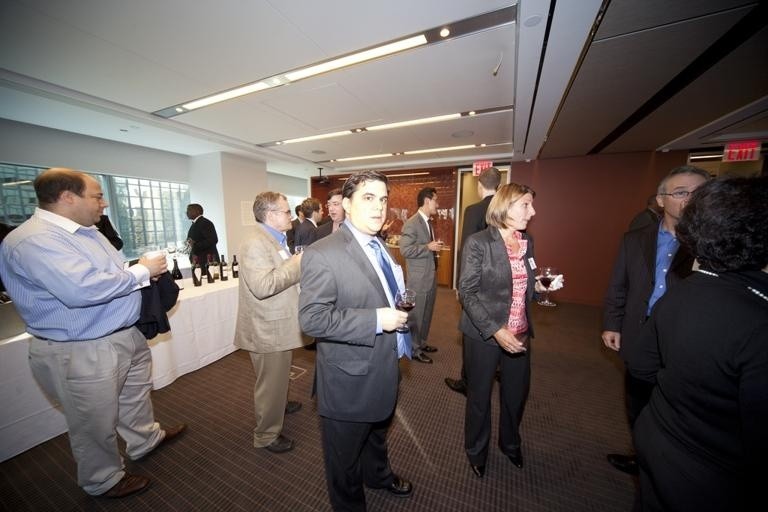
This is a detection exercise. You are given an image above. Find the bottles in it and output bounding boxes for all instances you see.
[171,255,239,290]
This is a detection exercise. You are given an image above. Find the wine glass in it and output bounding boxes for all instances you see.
[435,238,444,258]
[537,266,557,307]
[395,290,416,332]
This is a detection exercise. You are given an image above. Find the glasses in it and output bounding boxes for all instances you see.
[660,191,692,199]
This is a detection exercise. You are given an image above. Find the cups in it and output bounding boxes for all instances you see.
[294,244,308,255]
[144,251,168,258]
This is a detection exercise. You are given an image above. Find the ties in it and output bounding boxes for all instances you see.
[369,241,413,361]
[428,219,437,271]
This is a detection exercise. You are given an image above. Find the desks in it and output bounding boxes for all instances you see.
[0,270,238,465]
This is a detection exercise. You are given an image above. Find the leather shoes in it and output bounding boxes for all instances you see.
[99,473,152,499]
[412,353,433,363]
[266,433,294,453]
[472,462,485,478]
[608,454,637,474]
[367,473,413,498]
[508,450,523,468]
[285,400,302,414]
[445,377,467,394]
[422,345,438,352]
[161,423,186,442]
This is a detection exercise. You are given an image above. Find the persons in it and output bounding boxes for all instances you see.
[445,167,501,397]
[186,204,220,276]
[234,170,413,453]
[603,166,767,512]
[299,168,414,510]
[399,186,451,364]
[0,168,188,499]
[458,183,565,478]
